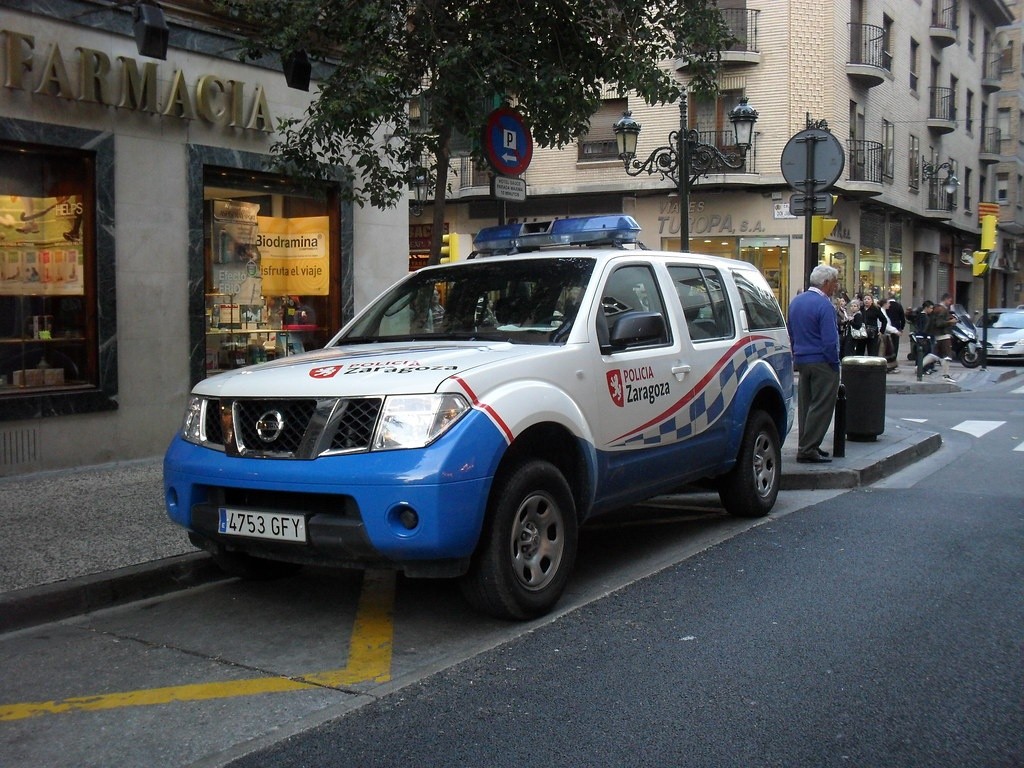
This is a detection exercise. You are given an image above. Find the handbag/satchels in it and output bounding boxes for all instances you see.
[850,311,868,339]
[866,326,878,337]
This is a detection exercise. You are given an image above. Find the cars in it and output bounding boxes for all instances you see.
[973,308,1024,366]
[163,216,796,624]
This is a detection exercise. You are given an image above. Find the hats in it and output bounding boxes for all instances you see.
[850,299,860,308]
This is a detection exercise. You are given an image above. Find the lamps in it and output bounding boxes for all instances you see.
[72,0,172,60]
[410,154,435,217]
[216,28,312,92]
[921,154,961,194]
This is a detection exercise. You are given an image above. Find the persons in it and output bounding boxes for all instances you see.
[242,296,285,325]
[905,301,934,373]
[785,265,842,463]
[932,293,959,382]
[831,291,904,374]
[410,286,573,333]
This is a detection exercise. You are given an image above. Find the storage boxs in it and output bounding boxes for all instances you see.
[45,315,56,338]
[206,351,219,370]
[25,315,44,339]
[13,368,64,385]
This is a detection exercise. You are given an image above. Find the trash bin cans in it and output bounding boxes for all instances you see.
[841,355,888,442]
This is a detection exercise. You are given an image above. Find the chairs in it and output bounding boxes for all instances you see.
[680,295,719,341]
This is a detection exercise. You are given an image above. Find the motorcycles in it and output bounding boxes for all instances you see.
[906,303,983,368]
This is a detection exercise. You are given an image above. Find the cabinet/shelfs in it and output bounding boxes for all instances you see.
[205,293,305,372]
[0,143,98,395]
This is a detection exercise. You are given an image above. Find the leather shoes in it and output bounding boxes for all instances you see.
[796,447,832,463]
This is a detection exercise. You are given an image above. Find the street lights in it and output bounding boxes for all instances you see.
[611,85,759,254]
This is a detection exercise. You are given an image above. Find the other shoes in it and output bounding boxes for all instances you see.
[926,371,930,374]
[942,375,956,383]
[915,366,924,376]
